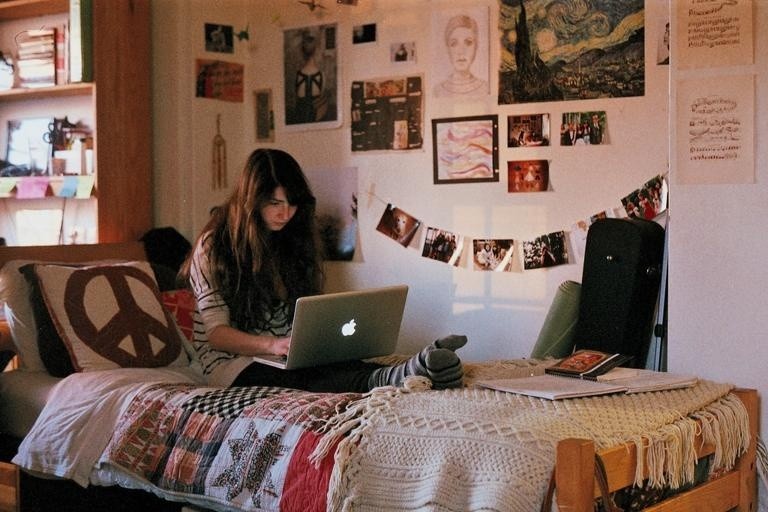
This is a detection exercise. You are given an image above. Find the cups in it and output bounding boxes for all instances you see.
[0,175,95,199]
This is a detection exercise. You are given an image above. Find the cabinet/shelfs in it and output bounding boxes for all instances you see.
[2,1,152,250]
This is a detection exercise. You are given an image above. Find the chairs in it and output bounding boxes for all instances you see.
[2,239,760,511]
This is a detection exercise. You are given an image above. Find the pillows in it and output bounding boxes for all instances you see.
[160,286,196,359]
[0,260,48,376]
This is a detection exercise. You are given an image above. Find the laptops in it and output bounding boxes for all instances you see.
[253,284,408,369]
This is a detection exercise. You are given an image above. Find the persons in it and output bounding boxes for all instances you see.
[538,240,556,266]
[658,21,670,64]
[434,15,488,95]
[178,149,467,390]
[474,243,506,269]
[560,114,603,145]
[626,181,667,219]
[296,37,326,122]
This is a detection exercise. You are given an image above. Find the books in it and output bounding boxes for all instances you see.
[475,370,695,401]
[544,349,637,381]
[17,0,93,87]
